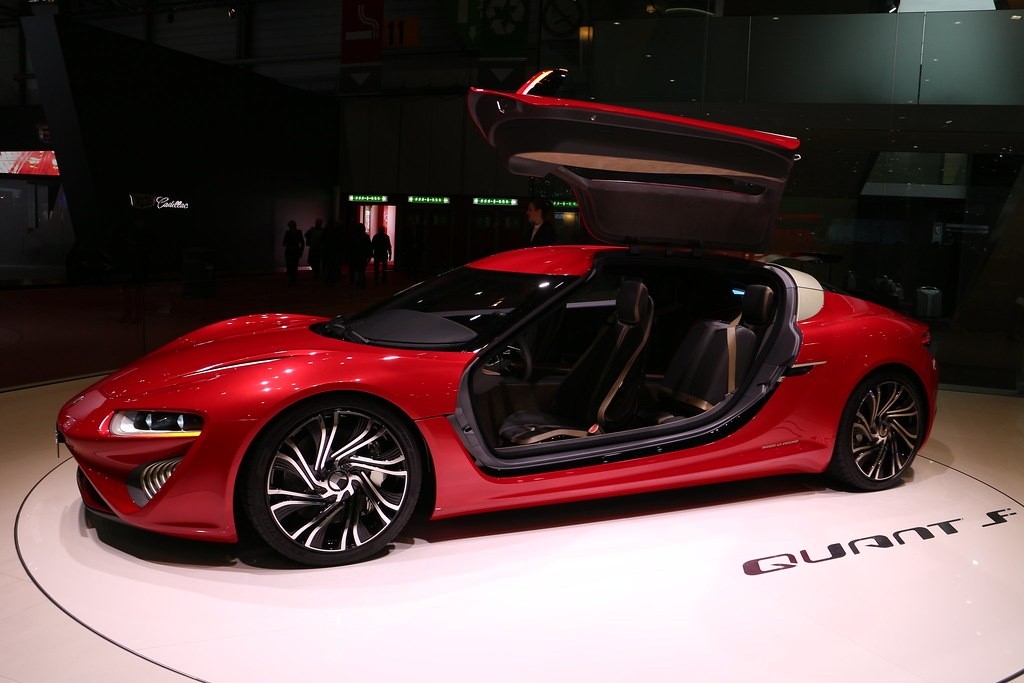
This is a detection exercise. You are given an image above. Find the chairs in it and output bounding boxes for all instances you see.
[667,282,787,416]
[496,282,653,446]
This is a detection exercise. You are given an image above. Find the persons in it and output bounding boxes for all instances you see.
[372,225,392,271]
[282,221,304,288]
[524,200,558,245]
[64,216,155,288]
[305,218,373,289]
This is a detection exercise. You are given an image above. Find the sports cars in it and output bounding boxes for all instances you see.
[55,66,941,570]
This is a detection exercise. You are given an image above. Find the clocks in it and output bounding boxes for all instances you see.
[543,1,584,36]
